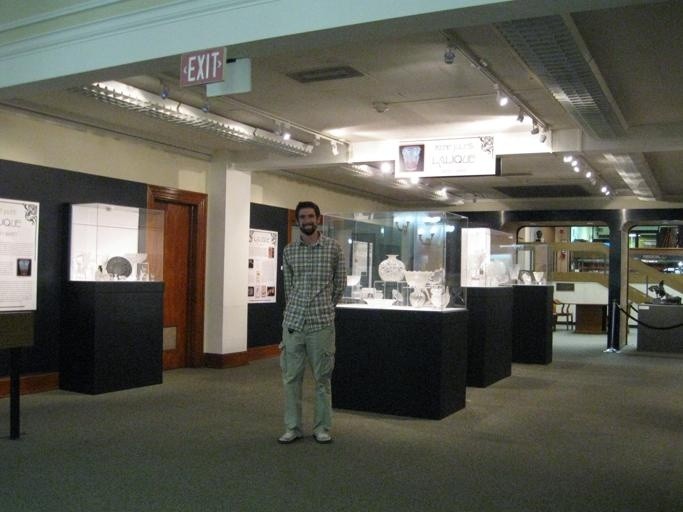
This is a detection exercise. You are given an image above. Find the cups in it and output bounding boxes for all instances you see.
[402,147,420,171]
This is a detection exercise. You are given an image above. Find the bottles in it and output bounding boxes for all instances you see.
[409,285,426,306]
[378,254,405,282]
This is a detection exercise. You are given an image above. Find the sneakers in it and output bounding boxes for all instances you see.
[277,430,303,444]
[313,428,332,443]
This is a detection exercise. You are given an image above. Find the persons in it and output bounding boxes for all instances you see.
[274,200,348,445]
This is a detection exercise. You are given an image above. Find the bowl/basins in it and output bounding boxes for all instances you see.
[402,271,431,287]
[347,275,361,286]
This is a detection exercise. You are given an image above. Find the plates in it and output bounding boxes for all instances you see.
[363,297,396,306]
